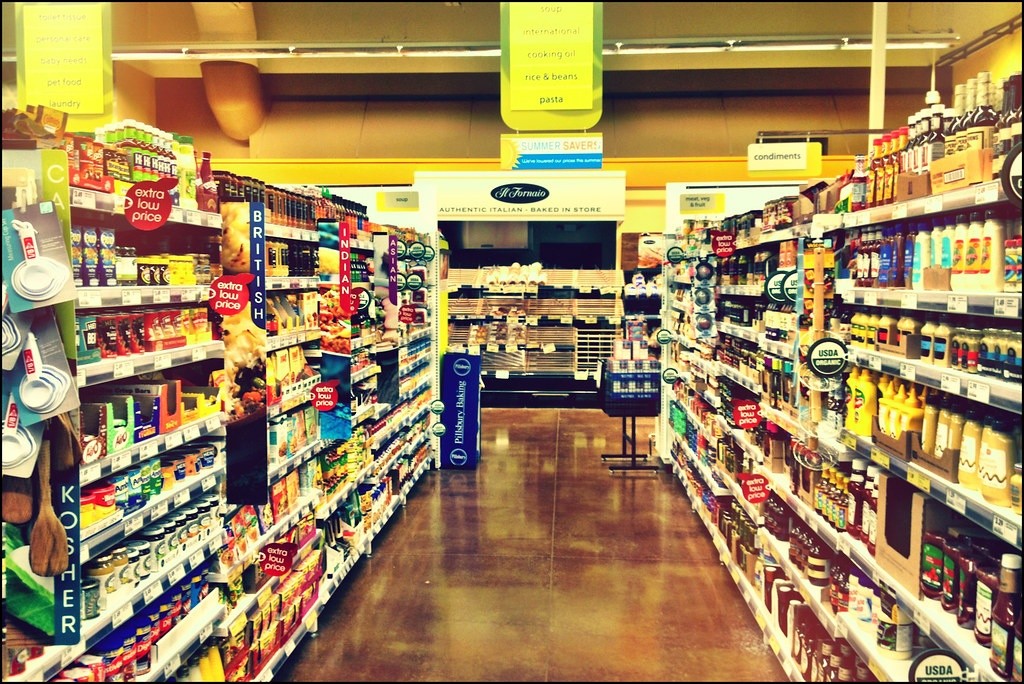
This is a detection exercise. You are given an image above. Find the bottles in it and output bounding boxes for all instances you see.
[165,635,227,682]
[666,71,1023,682]
[317,336,433,533]
[95,114,380,339]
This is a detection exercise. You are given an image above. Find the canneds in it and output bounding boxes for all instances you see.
[107,436,229,515]
[88,562,211,683]
[115,246,211,287]
[78,494,220,620]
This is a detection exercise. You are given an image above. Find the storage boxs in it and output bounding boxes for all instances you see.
[740,146,996,600]
[68,166,325,683]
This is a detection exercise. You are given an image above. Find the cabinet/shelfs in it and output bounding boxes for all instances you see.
[2,188,439,682]
[650,180,1023,683]
